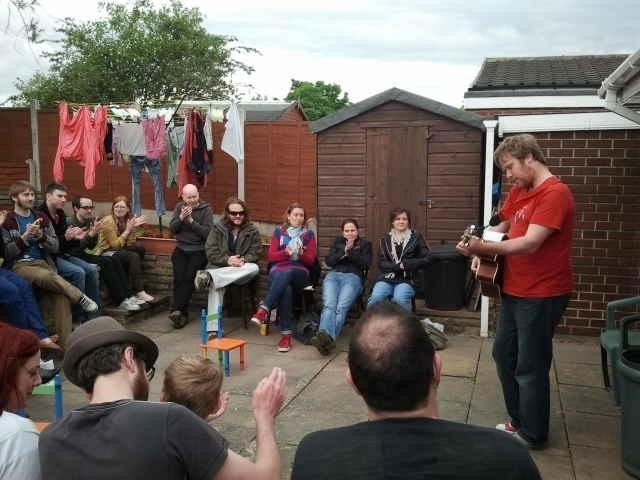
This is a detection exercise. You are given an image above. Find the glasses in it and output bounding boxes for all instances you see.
[22,364,40,378]
[135,352,155,382]
[79,206,94,210]
[113,205,126,209]
[226,209,245,216]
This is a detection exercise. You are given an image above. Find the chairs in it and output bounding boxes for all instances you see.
[599,296,640,406]
[14,374,63,433]
[199,303,247,377]
[224,278,258,329]
[265,259,317,335]
[320,267,368,317]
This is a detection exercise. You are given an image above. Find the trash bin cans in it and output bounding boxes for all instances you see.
[617,345,640,474]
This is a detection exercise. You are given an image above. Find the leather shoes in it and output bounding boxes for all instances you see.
[40,367,60,384]
[40,335,62,352]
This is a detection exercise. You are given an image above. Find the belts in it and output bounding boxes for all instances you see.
[16,258,35,262]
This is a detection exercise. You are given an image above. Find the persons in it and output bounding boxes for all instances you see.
[157,353,224,423]
[101,197,155,301]
[465,135,576,449]
[250,202,316,352]
[38,181,103,328]
[68,195,145,310]
[168,184,212,328]
[291,302,541,479]
[40,316,289,479]
[0,210,63,352]
[195,198,263,339]
[0,321,42,480]
[366,207,433,314]
[312,219,372,356]
[4,179,97,352]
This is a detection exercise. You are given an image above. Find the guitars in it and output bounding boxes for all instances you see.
[458,224,510,302]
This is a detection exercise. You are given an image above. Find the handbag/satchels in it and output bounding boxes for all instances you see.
[296,312,320,345]
[420,318,449,350]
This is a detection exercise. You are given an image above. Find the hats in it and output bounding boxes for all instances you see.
[63,316,159,388]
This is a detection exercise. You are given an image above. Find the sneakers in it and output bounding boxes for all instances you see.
[120,298,140,310]
[512,433,545,450]
[311,336,329,356]
[168,311,189,328]
[279,335,292,352]
[208,332,217,341]
[128,296,145,304]
[316,329,336,351]
[194,270,210,292]
[77,292,98,314]
[136,290,154,302]
[496,423,519,435]
[251,307,267,325]
